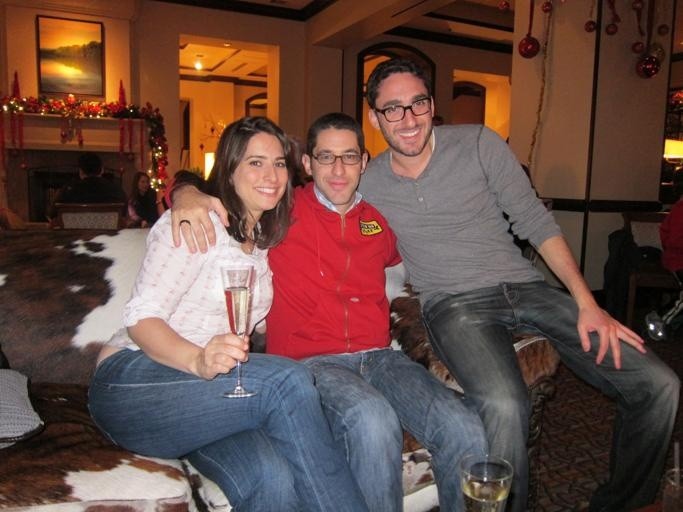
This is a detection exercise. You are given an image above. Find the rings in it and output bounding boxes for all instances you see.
[177,217,194,226]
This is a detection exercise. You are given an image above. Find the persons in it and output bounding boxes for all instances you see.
[125,172,165,227]
[50,154,126,220]
[352,55,682,512]
[169,113,487,512]
[88,115,370,512]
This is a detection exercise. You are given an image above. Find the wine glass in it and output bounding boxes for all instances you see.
[219,263,258,400]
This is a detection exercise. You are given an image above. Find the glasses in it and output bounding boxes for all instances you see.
[310,150,365,166]
[373,98,433,123]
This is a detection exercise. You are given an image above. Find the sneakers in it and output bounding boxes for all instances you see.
[645,310,669,342]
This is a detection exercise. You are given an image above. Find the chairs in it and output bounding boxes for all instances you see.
[57,203,126,231]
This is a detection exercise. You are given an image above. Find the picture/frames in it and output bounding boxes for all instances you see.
[35,14,105,98]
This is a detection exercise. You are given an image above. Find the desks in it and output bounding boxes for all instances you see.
[625,264,682,327]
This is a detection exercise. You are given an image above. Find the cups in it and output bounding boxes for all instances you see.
[658,468,683,512]
[459,455,515,512]
